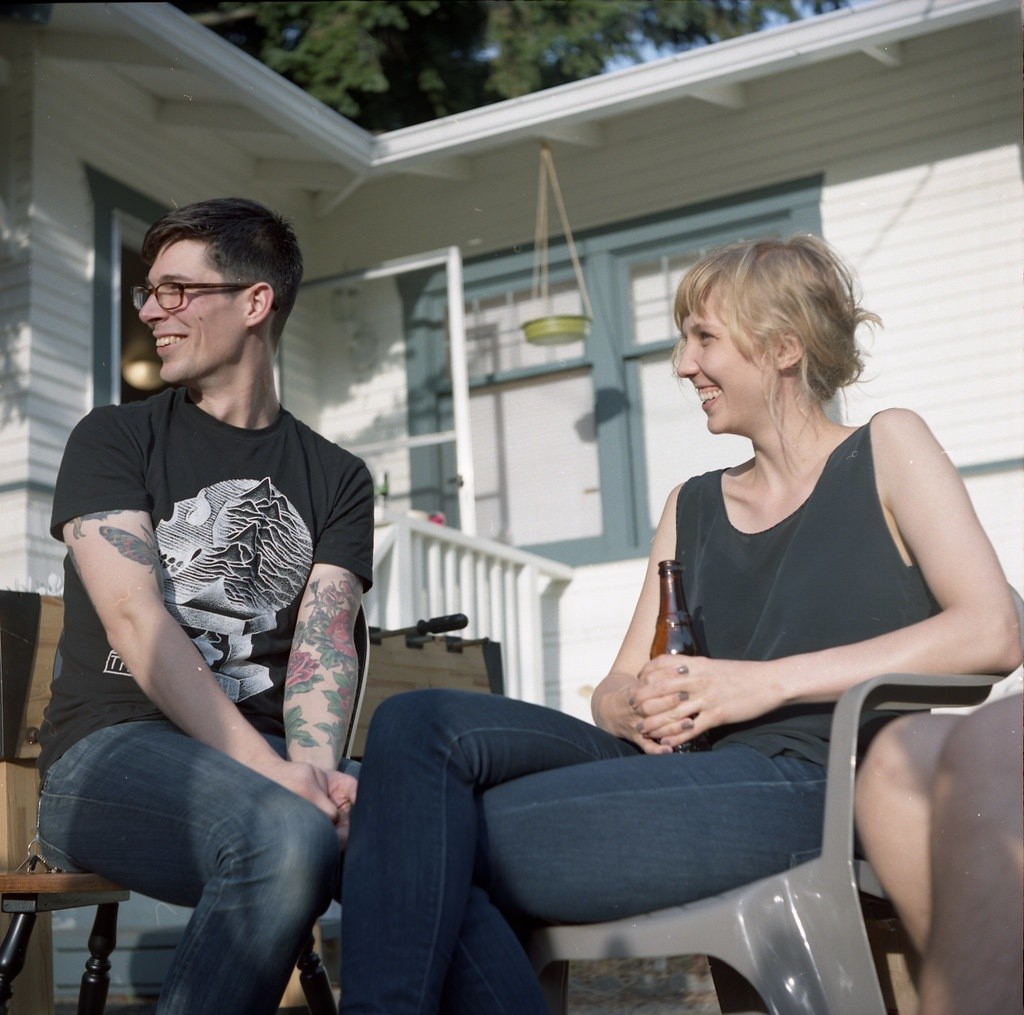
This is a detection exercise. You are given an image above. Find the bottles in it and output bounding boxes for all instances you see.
[649,559,713,753]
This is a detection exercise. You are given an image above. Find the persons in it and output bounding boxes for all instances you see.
[339,231,1024,1015]
[37,199,374,1015]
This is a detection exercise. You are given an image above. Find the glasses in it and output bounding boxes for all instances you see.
[129,282,281,311]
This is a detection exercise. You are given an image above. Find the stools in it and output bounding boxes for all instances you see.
[0,868,338,1015]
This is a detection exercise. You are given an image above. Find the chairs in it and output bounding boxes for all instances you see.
[524,583,1024,1015]
